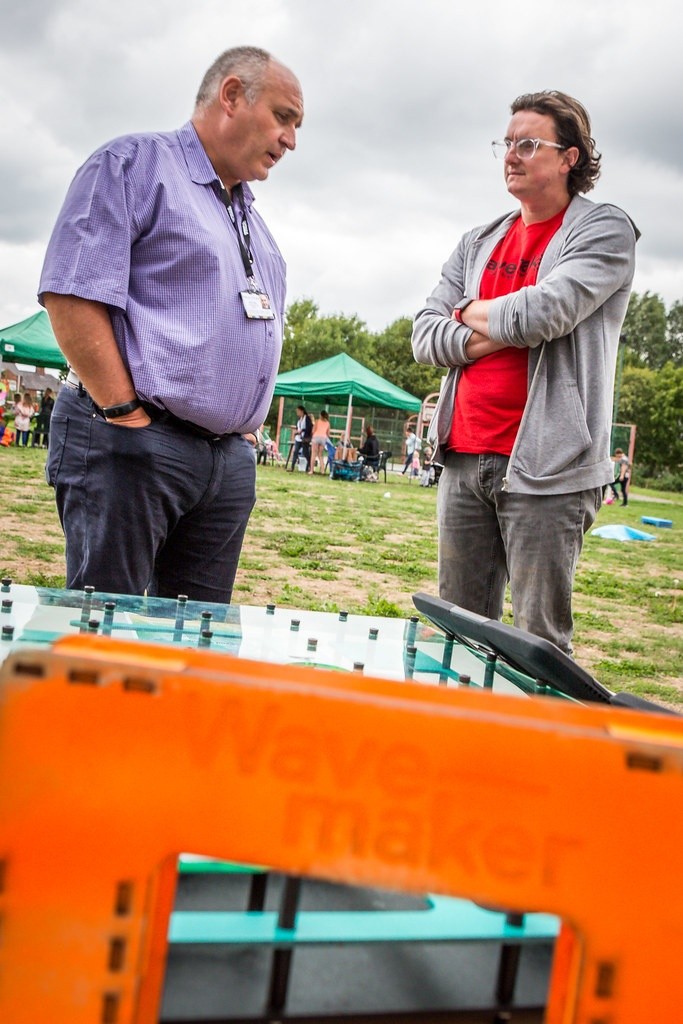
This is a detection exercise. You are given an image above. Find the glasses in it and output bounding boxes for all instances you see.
[491,137,566,160]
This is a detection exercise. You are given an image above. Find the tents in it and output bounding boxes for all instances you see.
[0,310,69,383]
[273,352,422,486]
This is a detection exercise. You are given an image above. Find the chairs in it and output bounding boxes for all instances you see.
[363,451,392,485]
[28,415,52,450]
[323,442,337,475]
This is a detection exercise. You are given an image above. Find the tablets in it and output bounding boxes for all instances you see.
[411,592,612,705]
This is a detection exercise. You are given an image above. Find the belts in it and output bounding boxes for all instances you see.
[141,402,220,441]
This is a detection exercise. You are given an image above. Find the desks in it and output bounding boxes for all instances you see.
[0,579,590,1024]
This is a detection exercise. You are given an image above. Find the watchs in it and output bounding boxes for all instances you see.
[453,298,475,324]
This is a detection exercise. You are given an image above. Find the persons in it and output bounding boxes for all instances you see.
[36,45,305,605]
[411,91,642,663]
[256,404,439,488]
[0,386,55,449]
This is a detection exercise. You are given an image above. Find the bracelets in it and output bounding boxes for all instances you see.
[91,396,142,419]
